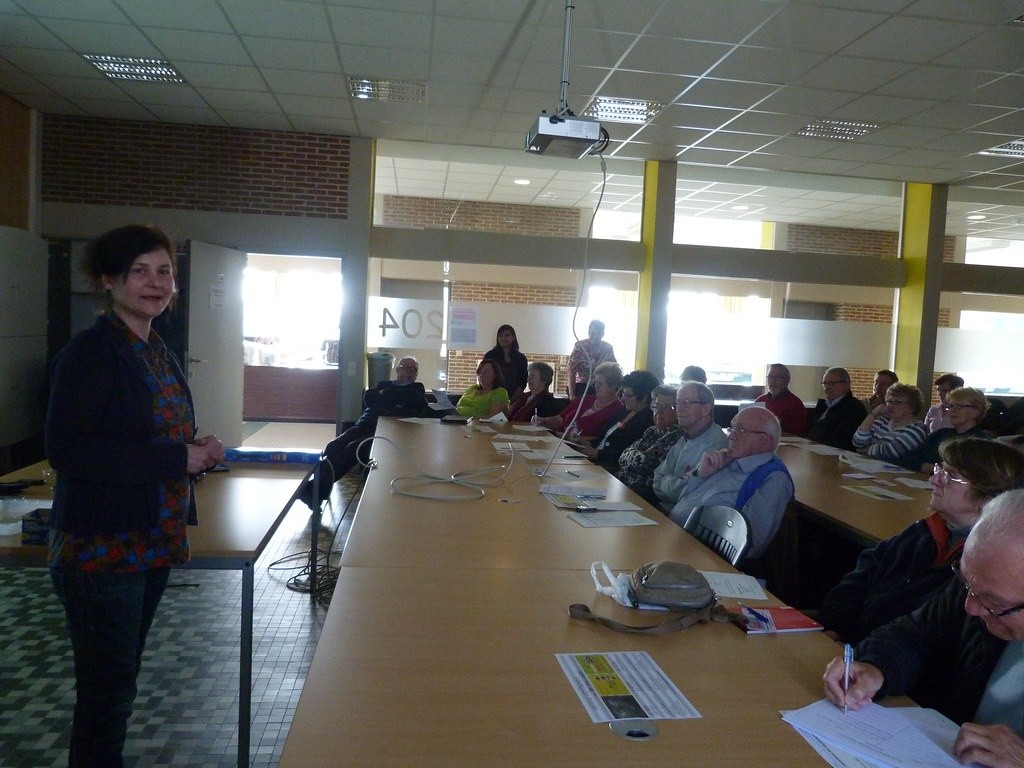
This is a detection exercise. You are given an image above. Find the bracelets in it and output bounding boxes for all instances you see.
[870,412,877,418]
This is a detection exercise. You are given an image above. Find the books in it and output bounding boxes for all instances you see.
[725,606,824,635]
[441,415,473,424]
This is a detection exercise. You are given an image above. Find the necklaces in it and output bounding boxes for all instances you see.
[594,399,602,411]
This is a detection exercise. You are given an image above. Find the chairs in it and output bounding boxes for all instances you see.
[683,504,754,567]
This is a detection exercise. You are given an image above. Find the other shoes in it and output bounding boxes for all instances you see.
[296,491,322,511]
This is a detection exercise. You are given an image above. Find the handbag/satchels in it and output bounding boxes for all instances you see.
[631,561,717,609]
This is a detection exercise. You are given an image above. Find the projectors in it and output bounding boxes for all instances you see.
[524,116,601,160]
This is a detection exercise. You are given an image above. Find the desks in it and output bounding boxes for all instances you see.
[369,417,596,466]
[277,567,926,768]
[346,463,740,574]
[721,428,946,561]
[0,447,324,768]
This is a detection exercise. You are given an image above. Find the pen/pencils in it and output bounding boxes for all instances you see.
[562,456,585,458]
[738,601,770,626]
[576,494,605,498]
[841,642,854,716]
[534,408,537,426]
[566,469,579,477]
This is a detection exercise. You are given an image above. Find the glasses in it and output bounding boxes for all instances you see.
[399,365,418,371]
[621,392,635,399]
[672,400,707,408]
[944,404,977,411]
[820,380,848,386]
[935,389,951,392]
[887,400,909,405]
[680,377,694,380]
[933,462,985,490]
[949,562,1024,617]
[727,427,769,435]
[766,376,787,380]
[650,403,673,410]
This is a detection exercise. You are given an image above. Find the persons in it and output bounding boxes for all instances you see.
[45,224,226,768]
[298,357,426,509]
[454,360,509,420]
[566,320,618,400]
[484,325,528,405]
[505,363,558,422]
[531,361,1024,768]
